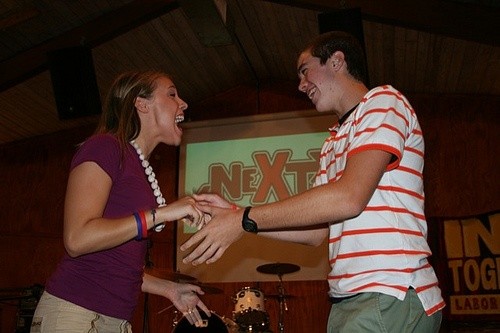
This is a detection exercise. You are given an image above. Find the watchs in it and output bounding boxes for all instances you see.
[242,206,258,233]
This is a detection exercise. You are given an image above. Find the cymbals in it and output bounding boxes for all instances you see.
[167,271,198,282]
[256,262,301,275]
[196,285,225,295]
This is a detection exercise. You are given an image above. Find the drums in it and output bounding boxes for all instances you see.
[231,287,269,328]
[172,308,230,333]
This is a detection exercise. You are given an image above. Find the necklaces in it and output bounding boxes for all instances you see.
[130,139,167,232]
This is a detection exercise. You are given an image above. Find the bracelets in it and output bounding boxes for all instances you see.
[133,209,148,241]
[150,206,157,228]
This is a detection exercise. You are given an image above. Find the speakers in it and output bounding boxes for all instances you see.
[46,42,104,121]
[318,7,370,87]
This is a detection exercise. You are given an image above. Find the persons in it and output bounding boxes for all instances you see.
[181,33,447,332]
[27,69,211,332]
